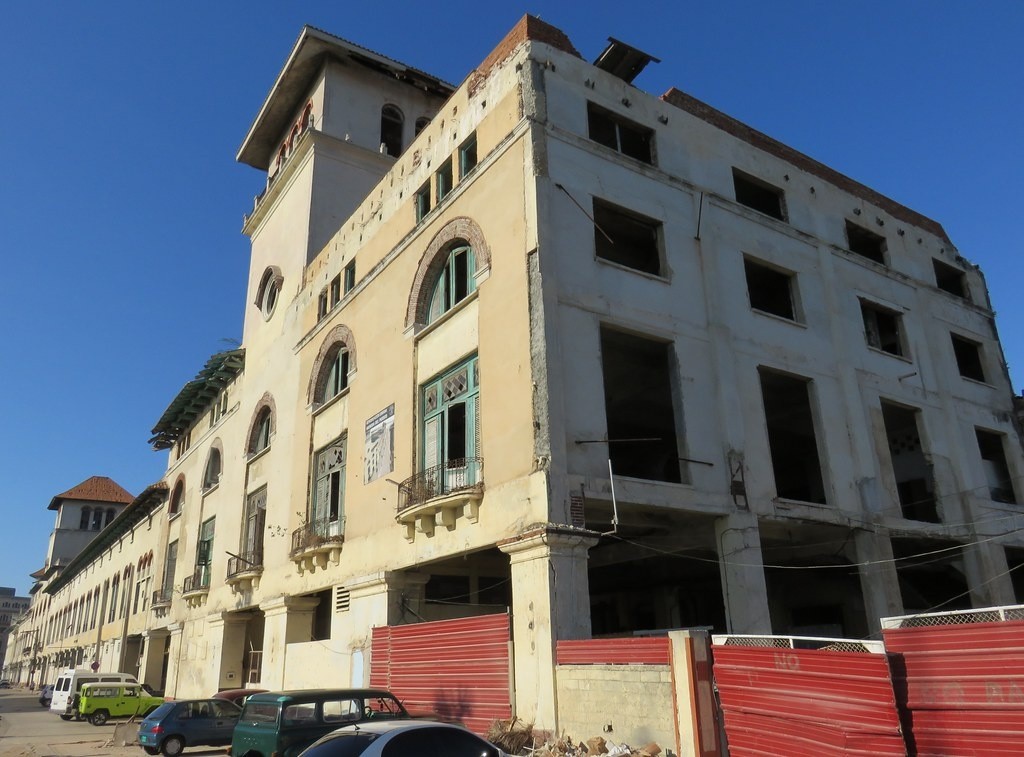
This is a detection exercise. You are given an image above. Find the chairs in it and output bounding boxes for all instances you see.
[199,705,208,719]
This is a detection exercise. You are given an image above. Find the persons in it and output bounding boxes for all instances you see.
[29,681,35,693]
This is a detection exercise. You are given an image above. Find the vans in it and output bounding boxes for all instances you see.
[49,668,152,721]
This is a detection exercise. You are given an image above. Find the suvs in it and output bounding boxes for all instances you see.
[70,682,167,726]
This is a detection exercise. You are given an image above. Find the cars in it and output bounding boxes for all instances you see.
[137,688,314,757]
[39,684,54,707]
[226,689,468,757]
[296,717,512,757]
